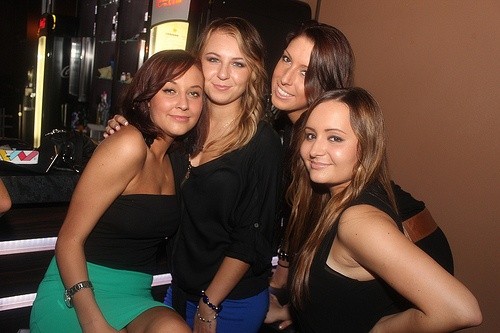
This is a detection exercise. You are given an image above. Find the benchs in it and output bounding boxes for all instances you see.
[0,235,174,311]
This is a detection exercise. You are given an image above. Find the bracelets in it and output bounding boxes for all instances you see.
[278,248,288,262]
[201,291,223,312]
[277,262,289,268]
[195,306,215,322]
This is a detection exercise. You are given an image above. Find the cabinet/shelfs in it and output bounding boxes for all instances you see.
[72,0,191,173]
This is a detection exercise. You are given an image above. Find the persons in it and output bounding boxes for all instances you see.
[31,50,210,333]
[0,177,12,213]
[281,86,483,333]
[102,17,285,333]
[264,22,454,333]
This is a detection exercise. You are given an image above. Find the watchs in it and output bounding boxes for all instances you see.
[65,280,92,307]
